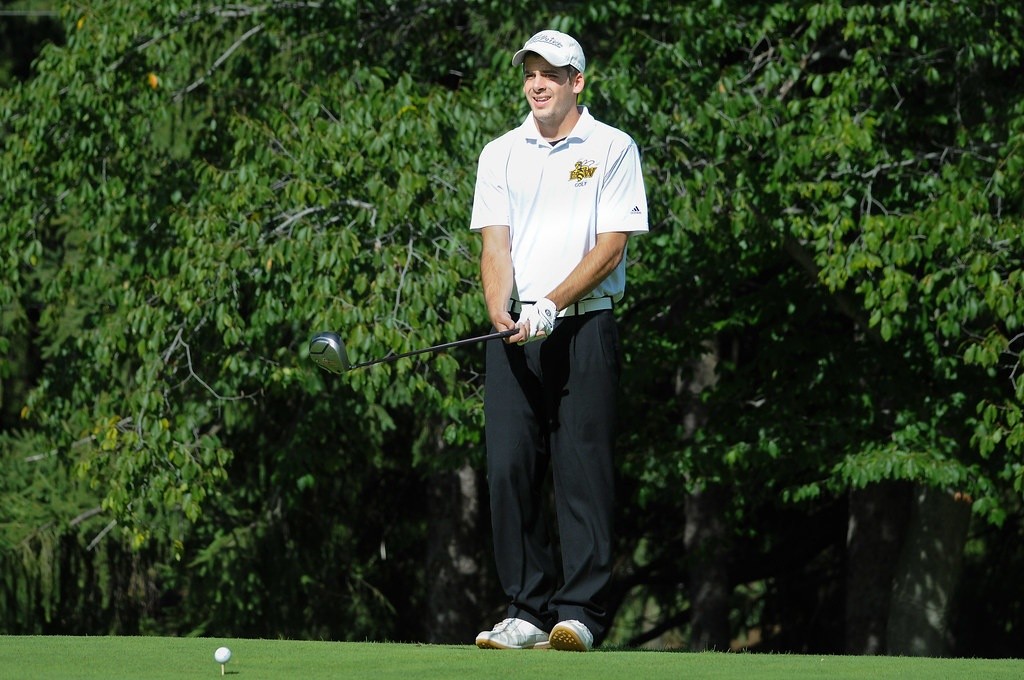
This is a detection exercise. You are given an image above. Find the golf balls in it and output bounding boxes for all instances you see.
[215,647,231,663]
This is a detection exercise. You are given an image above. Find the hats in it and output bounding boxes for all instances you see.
[512,30,586,74]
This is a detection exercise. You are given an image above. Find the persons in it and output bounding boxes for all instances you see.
[471,30,649,652]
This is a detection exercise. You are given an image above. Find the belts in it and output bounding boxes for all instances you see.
[507,298,613,318]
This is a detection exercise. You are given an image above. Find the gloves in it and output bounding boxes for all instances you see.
[514,298,559,345]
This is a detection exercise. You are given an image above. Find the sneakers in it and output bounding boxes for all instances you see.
[549,619,594,651]
[475,617,550,650]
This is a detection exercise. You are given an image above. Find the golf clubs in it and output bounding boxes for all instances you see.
[307,327,521,375]
